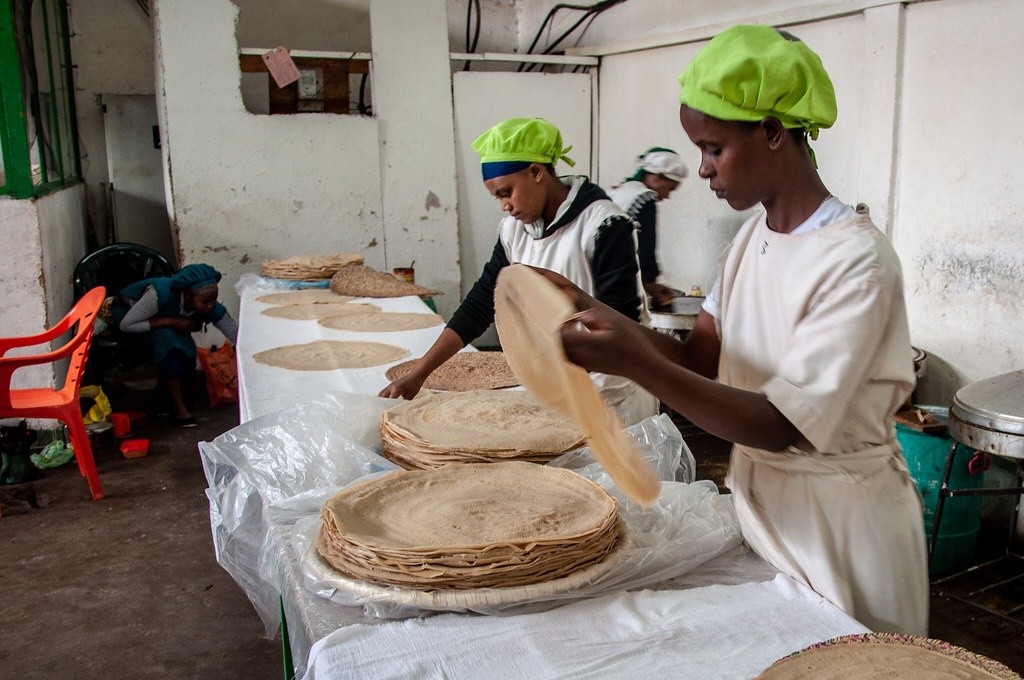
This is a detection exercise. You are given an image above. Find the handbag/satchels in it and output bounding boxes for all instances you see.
[195,340,239,405]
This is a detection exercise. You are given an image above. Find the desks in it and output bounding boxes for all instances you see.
[236,275,1024,680]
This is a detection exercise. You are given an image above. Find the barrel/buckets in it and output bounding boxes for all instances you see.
[894,404,984,558]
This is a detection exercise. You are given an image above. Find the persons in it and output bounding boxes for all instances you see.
[607,143,687,313]
[512,22,930,643]
[98,264,236,439]
[381,117,672,430]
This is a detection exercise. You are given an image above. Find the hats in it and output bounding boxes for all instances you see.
[470,116,576,169]
[678,24,837,170]
[173,262,222,292]
[638,148,689,184]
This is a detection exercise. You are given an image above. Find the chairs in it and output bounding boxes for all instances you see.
[70,242,178,415]
[0,286,106,499]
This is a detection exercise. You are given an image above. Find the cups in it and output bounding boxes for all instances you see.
[394,268,414,284]
